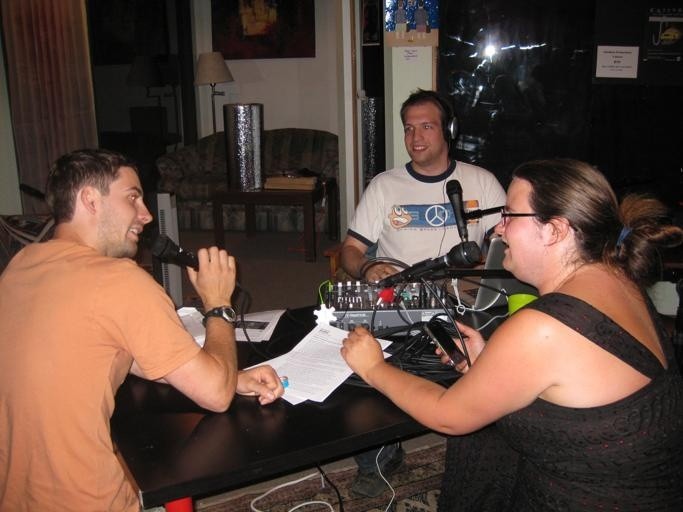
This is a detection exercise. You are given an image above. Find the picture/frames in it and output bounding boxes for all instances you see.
[210,1,315,58]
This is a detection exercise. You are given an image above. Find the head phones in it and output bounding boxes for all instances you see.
[406,92,458,139]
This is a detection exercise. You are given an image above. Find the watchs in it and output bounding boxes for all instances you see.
[203,306,237,327]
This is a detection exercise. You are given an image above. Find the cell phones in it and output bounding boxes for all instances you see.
[423,321,466,368]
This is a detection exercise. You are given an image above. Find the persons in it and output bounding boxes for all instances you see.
[339,90,507,282]
[341,160,683,512]
[0,150,284,512]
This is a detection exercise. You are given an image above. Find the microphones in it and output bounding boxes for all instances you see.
[151,234,242,288]
[380,240,481,286]
[446,179,469,243]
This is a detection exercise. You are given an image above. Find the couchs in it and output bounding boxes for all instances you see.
[154,127,339,232]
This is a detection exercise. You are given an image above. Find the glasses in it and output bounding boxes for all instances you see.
[500,208,538,226]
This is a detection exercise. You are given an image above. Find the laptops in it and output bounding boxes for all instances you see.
[433,237,539,311]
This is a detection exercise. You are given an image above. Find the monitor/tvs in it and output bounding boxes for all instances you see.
[137,189,183,310]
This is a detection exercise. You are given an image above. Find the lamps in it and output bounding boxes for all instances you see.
[193,51,235,133]
[125,54,166,108]
